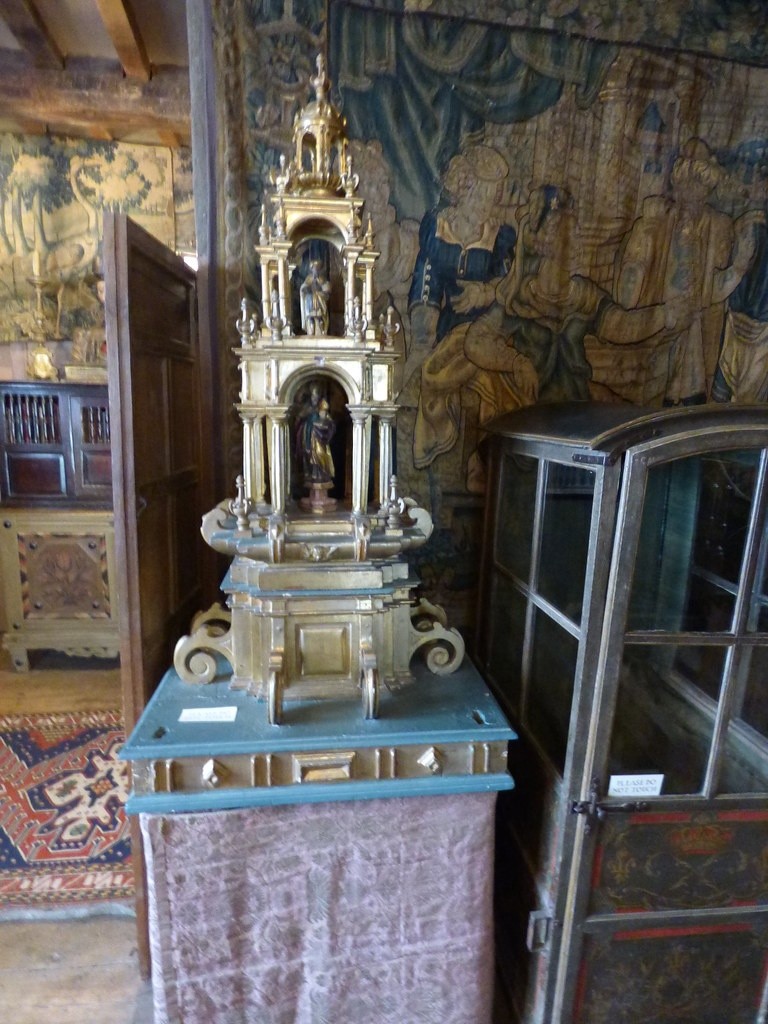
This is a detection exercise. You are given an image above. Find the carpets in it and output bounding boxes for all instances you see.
[0,708,135,923]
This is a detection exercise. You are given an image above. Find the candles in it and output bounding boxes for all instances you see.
[32,251,41,277]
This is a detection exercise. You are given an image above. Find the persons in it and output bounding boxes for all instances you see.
[299,259,332,336]
[293,384,335,483]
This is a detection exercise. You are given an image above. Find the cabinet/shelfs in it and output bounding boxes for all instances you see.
[1,379,121,674]
